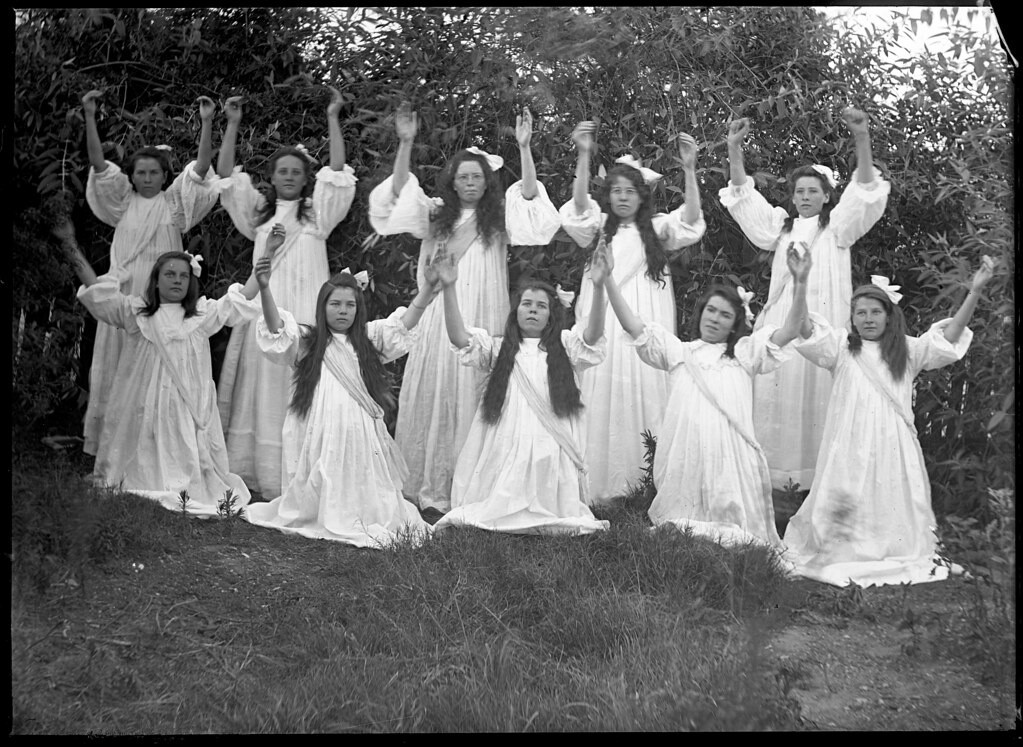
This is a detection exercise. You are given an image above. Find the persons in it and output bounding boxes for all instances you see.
[559,121,708,505]
[80,89,219,479]
[434,242,609,536]
[220,85,358,499]
[598,229,815,576]
[787,241,993,586]
[50,217,287,520]
[241,243,445,546]
[719,109,891,490]
[368,99,561,515]
